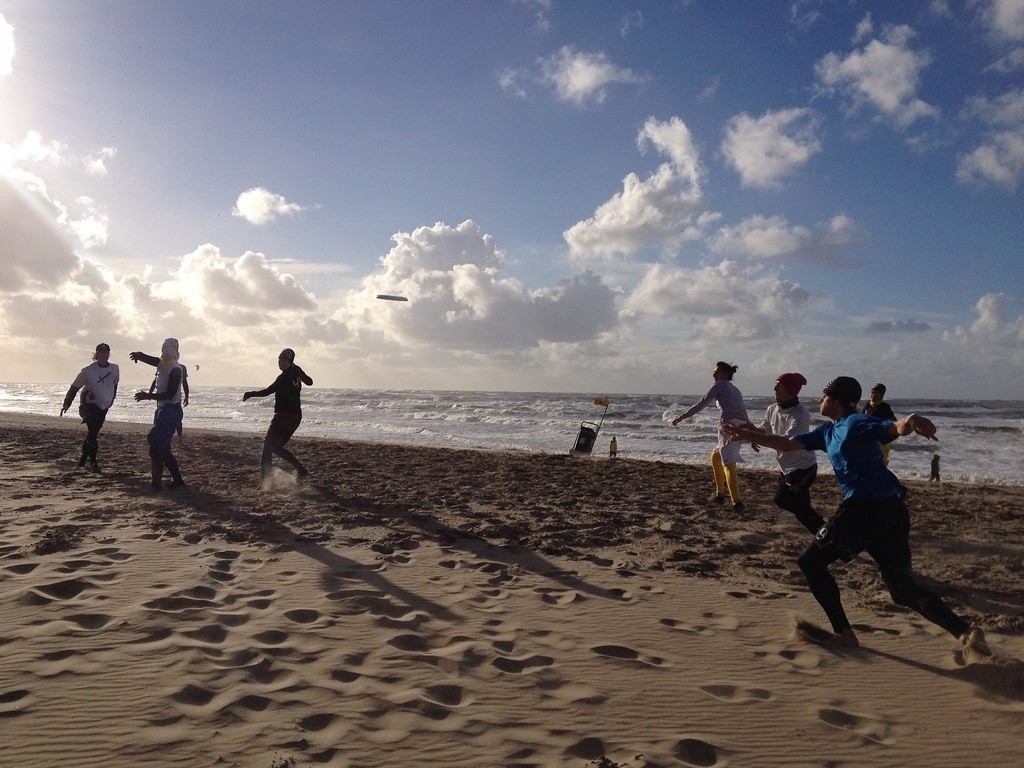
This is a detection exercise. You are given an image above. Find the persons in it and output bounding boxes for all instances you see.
[719,376,993,661]
[130,337,186,494]
[147,350,189,448]
[672,361,761,512]
[928,453,941,482]
[61,343,120,477]
[609,436,617,458]
[725,373,826,537]
[861,382,898,469]
[243,348,313,494]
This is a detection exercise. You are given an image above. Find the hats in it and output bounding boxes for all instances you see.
[872,384,886,396]
[162,338,179,350]
[823,377,862,410]
[777,373,807,396]
[97,343,110,351]
[280,349,295,362]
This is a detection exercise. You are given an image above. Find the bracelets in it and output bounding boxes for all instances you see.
[252,391,256,397]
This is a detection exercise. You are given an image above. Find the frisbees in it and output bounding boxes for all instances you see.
[377,295,408,301]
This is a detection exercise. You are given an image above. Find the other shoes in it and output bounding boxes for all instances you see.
[709,496,725,504]
[731,503,743,510]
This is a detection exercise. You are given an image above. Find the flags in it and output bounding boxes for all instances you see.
[592,397,609,406]
